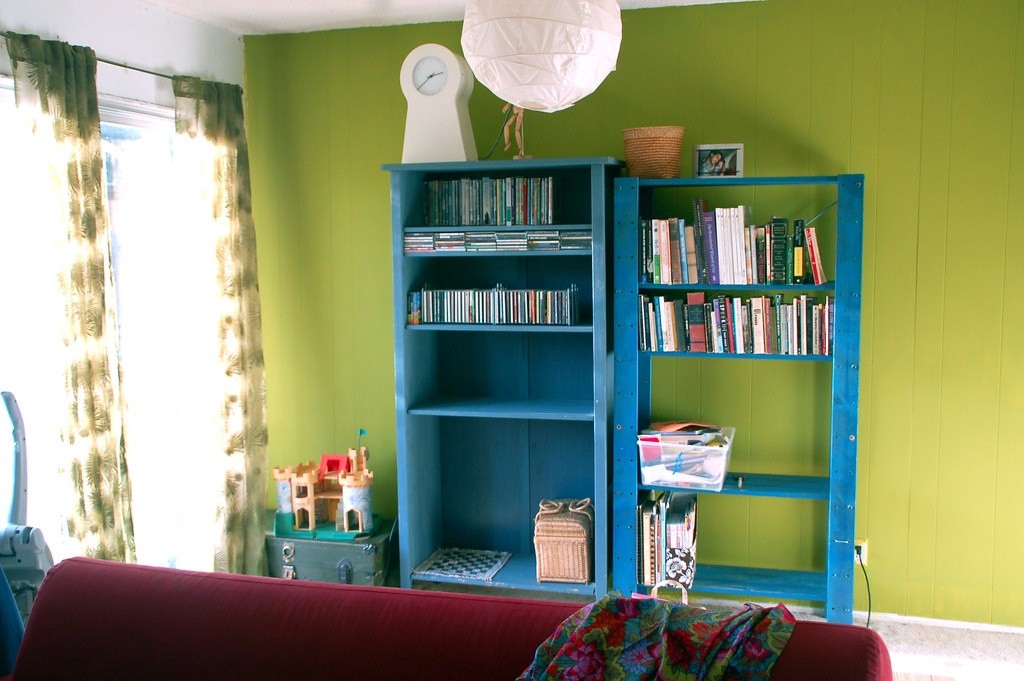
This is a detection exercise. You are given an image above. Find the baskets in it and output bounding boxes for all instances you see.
[622,125,686,177]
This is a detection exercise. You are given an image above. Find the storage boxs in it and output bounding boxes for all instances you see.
[637,426,736,491]
[262,509,397,587]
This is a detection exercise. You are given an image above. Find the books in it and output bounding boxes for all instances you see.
[640,490,698,588]
[407,283,578,325]
[422,176,554,225]
[403,230,591,252]
[639,197,828,286]
[638,291,833,356]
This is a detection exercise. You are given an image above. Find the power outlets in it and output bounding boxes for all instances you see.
[855,539,868,564]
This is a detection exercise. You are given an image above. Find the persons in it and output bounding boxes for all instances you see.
[698,152,726,176]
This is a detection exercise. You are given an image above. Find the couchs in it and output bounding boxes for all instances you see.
[0,557,892,681]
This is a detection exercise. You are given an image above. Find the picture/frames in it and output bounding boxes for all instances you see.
[692,144,743,179]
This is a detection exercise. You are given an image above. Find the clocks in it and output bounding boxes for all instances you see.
[400,42,479,162]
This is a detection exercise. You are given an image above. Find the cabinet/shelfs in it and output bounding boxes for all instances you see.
[381,158,627,601]
[612,173,864,627]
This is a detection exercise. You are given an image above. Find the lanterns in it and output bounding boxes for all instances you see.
[460,0,623,114]
